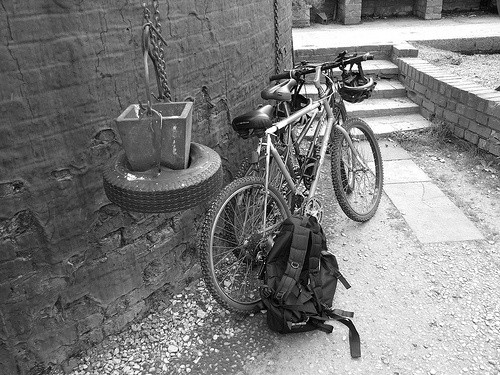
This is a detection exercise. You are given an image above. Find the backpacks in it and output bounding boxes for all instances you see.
[257,216,362,359]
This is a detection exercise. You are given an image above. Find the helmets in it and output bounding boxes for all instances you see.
[276,92,313,116]
[337,72,377,103]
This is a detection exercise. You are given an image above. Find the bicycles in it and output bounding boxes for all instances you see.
[225,63,356,266]
[200,50,384,316]
